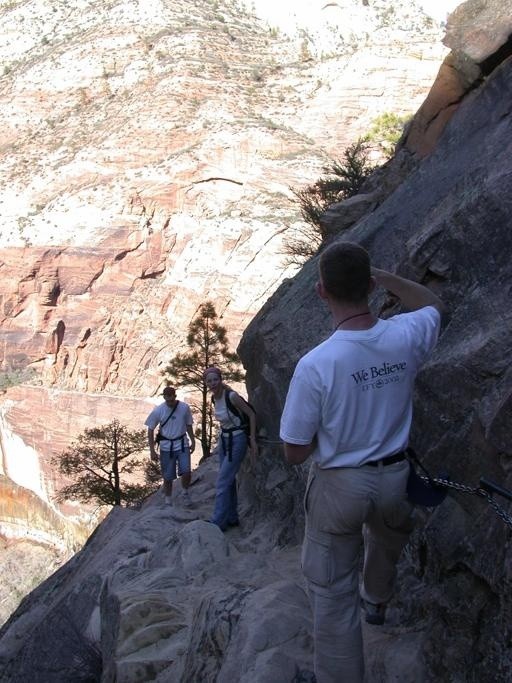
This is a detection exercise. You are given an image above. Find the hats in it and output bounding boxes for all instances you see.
[160,387,175,395]
[203,367,221,377]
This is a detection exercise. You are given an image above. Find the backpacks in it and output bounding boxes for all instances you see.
[211,389,257,435]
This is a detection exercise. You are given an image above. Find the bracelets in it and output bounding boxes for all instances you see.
[249,435,256,439]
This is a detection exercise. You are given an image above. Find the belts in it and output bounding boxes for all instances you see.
[365,450,407,468]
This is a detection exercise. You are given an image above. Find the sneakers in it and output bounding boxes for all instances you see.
[203,518,239,533]
[360,597,388,626]
[181,493,193,509]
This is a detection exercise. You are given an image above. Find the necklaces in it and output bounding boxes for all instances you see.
[333,310,371,330]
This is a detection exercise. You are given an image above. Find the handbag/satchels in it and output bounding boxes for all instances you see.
[155,430,167,446]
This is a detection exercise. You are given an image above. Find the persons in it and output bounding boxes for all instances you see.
[202,365,260,532]
[276,239,450,680]
[142,386,196,506]
